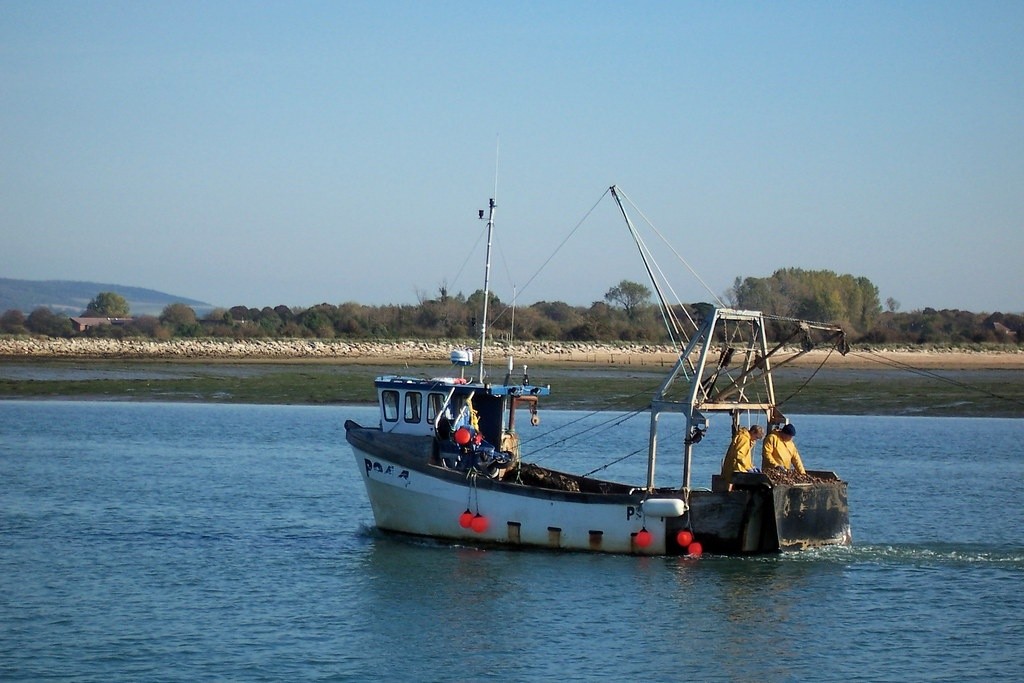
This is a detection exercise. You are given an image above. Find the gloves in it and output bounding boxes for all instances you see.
[775,466,786,473]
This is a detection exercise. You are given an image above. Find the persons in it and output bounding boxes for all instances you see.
[721,424,764,492]
[762,424,806,475]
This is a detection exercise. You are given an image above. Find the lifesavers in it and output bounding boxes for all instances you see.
[432,377,467,385]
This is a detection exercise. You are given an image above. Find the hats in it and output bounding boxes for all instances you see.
[782,424,796,436]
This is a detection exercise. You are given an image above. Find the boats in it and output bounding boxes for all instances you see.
[450,343,474,366]
[342,129,854,556]
